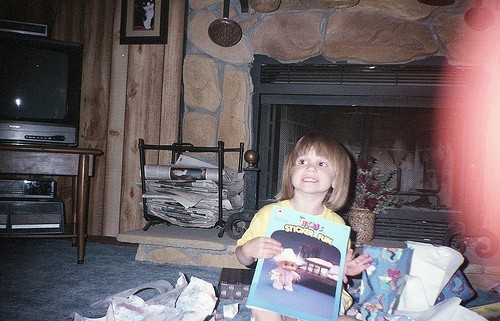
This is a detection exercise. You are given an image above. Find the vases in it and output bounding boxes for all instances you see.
[347,207,375,244]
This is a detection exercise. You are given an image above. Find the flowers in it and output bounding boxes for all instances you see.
[348,154,394,212]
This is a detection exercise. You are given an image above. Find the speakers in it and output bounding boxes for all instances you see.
[0,31,83,147]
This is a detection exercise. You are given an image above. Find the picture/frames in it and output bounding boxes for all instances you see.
[119,0,170,45]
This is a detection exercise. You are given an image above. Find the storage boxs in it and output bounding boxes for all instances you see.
[218,267,256,300]
[0,179,69,234]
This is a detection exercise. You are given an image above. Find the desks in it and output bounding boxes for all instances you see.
[0,146,104,266]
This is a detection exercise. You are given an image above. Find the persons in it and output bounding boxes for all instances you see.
[234,132,372,320]
